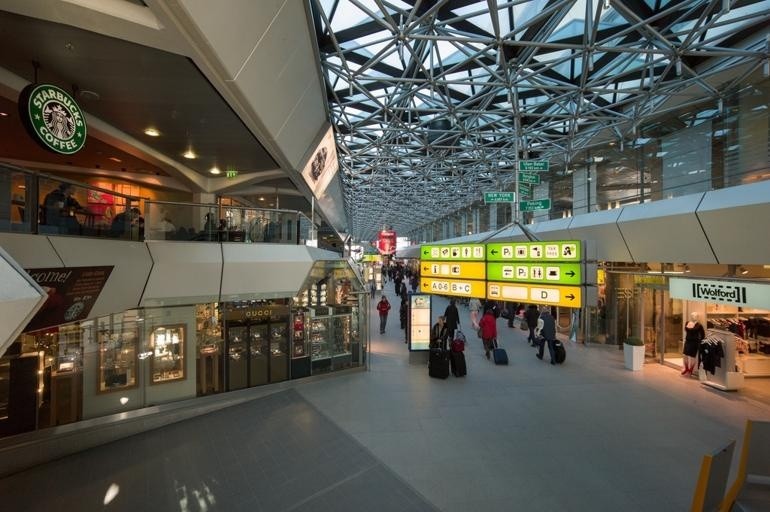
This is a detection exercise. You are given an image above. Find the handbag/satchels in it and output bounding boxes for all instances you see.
[489,340,497,349]
[452,339,465,352]
[520,318,528,330]
[501,310,511,319]
[537,312,544,330]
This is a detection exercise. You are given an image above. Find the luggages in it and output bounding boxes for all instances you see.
[457,324,466,343]
[449,348,467,377]
[492,338,509,365]
[428,338,449,380]
[551,339,566,364]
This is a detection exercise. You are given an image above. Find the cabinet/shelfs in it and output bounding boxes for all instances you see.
[291,305,353,374]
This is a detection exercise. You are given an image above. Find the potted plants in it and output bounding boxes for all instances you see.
[623,336,646,371]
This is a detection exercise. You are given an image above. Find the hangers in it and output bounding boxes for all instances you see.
[707,314,745,331]
[700,332,725,348]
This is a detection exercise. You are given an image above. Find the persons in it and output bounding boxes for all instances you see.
[483,300,500,319]
[54,181,86,234]
[111,207,142,239]
[432,315,449,352]
[478,306,496,360]
[39,189,67,227]
[535,309,556,365]
[380,262,421,297]
[369,279,377,298]
[130,212,241,244]
[468,297,483,330]
[679,312,705,377]
[443,298,460,340]
[376,295,391,336]
[524,306,540,347]
[506,302,518,328]
[400,298,409,344]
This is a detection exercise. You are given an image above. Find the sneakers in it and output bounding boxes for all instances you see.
[485,350,490,360]
[380,330,386,336]
[536,353,543,360]
[528,337,537,348]
[508,325,516,328]
